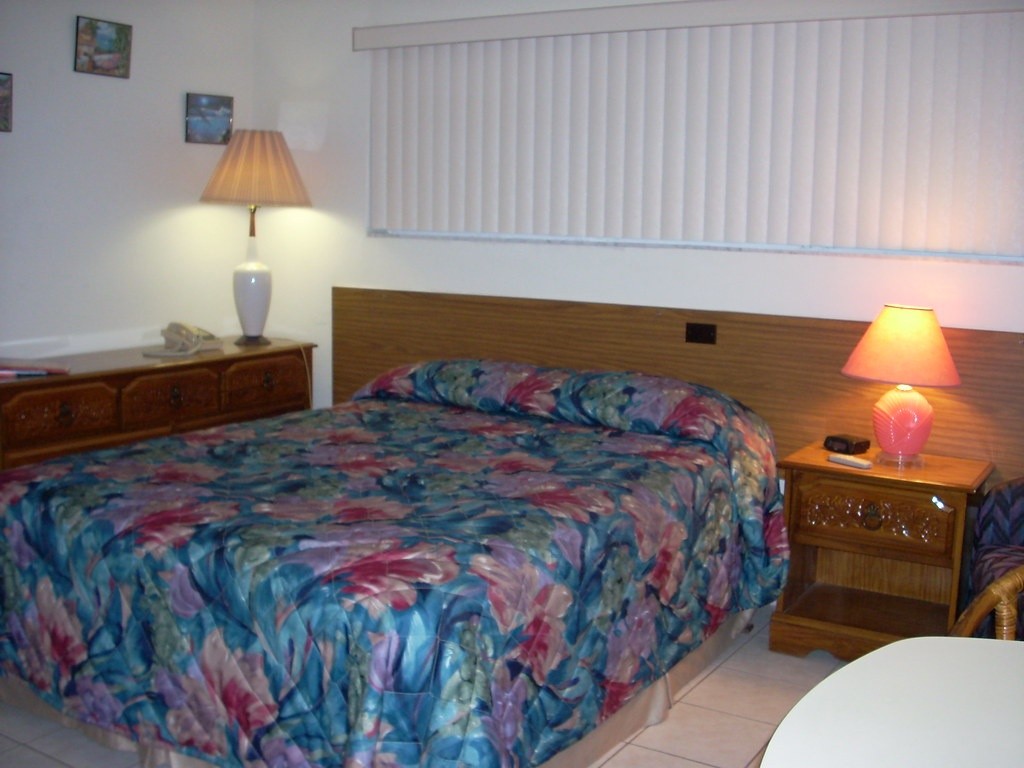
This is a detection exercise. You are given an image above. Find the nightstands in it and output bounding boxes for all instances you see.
[769,440,996,663]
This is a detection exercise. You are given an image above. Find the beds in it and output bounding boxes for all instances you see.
[0,358,792,768]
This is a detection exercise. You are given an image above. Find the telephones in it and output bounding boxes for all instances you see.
[160,320,224,351]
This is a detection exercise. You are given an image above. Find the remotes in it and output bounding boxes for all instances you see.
[828,454,872,468]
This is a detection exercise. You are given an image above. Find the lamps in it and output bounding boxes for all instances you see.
[839,305,962,471]
[198,130,312,337]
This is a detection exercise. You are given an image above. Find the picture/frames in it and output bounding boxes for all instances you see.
[74,16,133,79]
[0,72,12,132]
[186,92,233,145]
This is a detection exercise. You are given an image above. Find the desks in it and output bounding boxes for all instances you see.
[0,335,317,472]
[759,635,1024,768]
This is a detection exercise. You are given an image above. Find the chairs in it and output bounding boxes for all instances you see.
[953,477,1024,642]
